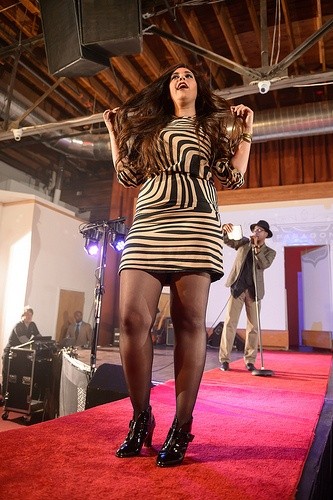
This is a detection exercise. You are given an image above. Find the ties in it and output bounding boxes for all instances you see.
[75,325,80,338]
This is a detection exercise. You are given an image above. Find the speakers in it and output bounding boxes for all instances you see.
[85,363,153,408]
[39,0,110,78]
[210,321,246,352]
[77,0,143,58]
[166,318,175,346]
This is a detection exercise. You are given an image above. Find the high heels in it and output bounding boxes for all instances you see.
[114,406,155,458]
[155,414,194,467]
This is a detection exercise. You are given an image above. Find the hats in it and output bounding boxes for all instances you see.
[251,220,274,238]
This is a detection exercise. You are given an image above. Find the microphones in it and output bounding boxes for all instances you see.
[249,239,254,246]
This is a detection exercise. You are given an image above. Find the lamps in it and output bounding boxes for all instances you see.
[105,218,129,251]
[85,225,103,256]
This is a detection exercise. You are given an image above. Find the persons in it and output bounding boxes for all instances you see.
[66,311,93,347]
[102,63,255,466]
[1,306,42,404]
[218,220,277,373]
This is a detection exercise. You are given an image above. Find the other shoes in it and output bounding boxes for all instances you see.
[246,363,256,371]
[220,362,229,371]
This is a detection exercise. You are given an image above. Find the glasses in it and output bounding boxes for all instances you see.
[252,228,265,232]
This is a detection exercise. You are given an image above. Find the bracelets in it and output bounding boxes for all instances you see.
[254,245,260,249]
[241,133,253,143]
